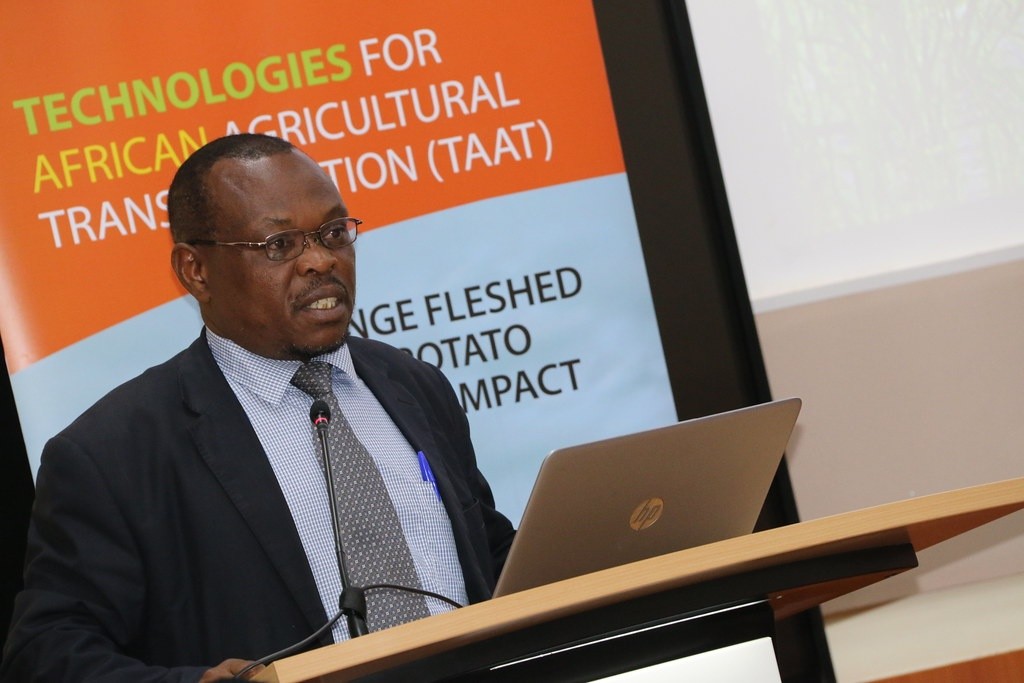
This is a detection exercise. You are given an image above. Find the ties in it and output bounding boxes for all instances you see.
[290,362,431,633]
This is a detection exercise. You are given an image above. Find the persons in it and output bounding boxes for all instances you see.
[1,130,521,682]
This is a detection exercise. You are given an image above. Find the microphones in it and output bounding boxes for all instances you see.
[310,397,371,640]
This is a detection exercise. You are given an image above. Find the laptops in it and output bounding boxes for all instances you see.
[486,396,804,607]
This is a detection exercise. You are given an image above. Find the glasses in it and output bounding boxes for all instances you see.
[184,217,362,261]
